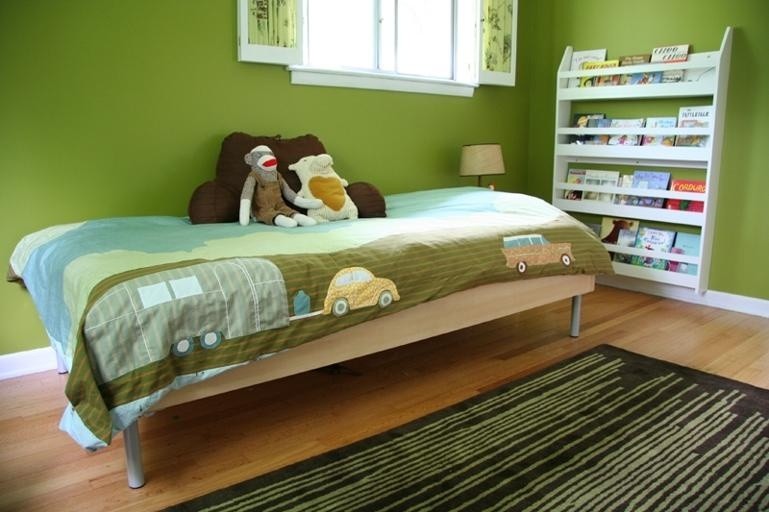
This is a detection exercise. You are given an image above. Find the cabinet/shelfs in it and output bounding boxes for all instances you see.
[552,26,733,294]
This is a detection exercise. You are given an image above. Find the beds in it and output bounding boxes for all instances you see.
[8,186,614,488]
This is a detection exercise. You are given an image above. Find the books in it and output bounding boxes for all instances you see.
[569,106,712,148]
[586,217,700,276]
[564,168,706,212]
[568,44,689,87]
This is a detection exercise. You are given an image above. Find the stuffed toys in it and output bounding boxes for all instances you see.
[288,154,359,223]
[239,145,323,228]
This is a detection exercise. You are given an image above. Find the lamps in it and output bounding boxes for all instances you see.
[459,144,506,187]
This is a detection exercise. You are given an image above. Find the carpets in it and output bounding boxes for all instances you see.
[160,343,769,511]
